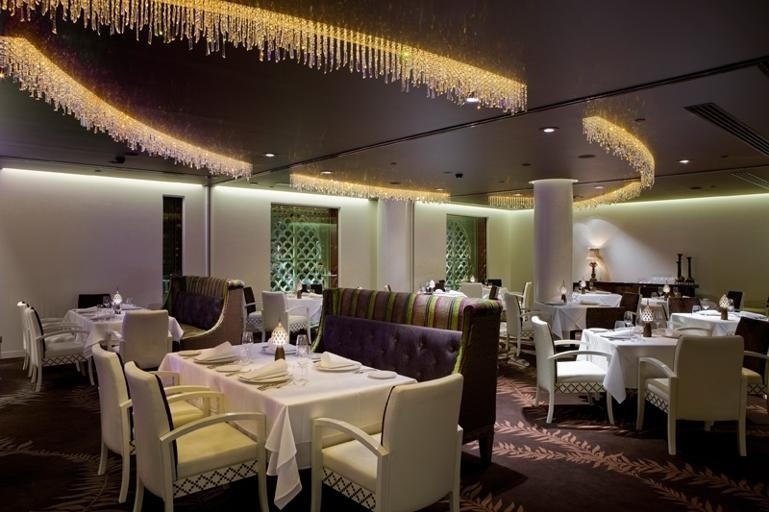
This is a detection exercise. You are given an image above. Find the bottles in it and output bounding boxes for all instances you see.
[274,343,286,362]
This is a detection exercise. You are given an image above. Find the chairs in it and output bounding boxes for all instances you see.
[89,340,212,505]
[112,308,174,370]
[259,290,312,348]
[122,358,273,512]
[14,299,95,394]
[309,372,466,512]
[239,285,263,334]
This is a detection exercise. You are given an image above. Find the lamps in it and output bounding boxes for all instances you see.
[585,248,602,282]
[113,293,123,315]
[269,321,290,361]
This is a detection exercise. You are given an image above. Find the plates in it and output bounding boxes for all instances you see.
[263,344,298,354]
[543,301,565,306]
[602,333,635,340]
[590,328,608,333]
[193,355,240,364]
[317,358,362,372]
[237,370,291,384]
[369,369,398,379]
[216,364,240,372]
[178,350,203,357]
[580,301,600,305]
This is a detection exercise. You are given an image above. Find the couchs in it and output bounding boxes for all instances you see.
[154,272,247,352]
[307,286,504,469]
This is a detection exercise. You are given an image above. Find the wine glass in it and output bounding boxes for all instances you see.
[96,296,134,320]
[242,330,255,364]
[589,281,593,293]
[651,287,678,300]
[615,311,646,343]
[296,336,309,384]
[692,299,735,319]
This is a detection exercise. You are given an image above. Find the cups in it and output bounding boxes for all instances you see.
[444,287,451,294]
[287,289,315,299]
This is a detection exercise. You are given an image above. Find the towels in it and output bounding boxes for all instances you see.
[198,339,238,362]
[245,358,290,381]
[320,351,356,369]
